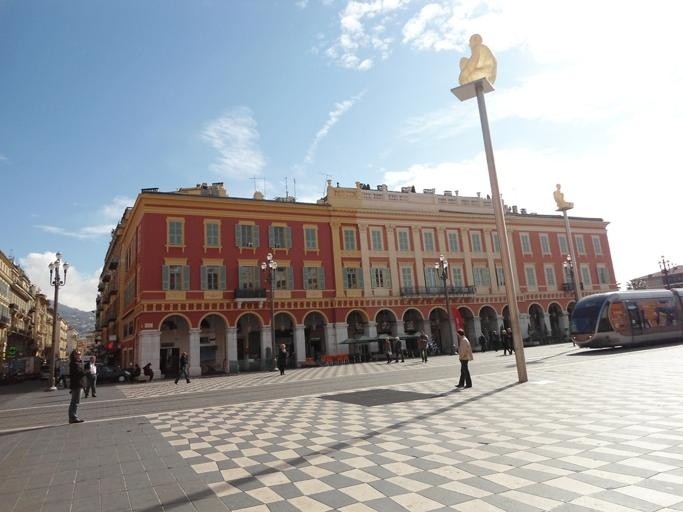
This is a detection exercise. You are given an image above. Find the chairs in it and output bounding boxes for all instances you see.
[321,354,351,366]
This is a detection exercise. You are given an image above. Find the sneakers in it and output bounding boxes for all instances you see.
[85,393,96,397]
[457,385,472,388]
[70,418,83,423]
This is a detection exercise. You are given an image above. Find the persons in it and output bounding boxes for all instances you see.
[417,329,429,363]
[277,344,287,375]
[55,365,67,388]
[478,326,516,356]
[395,336,404,362]
[174,352,191,384]
[84,357,97,398]
[68,350,91,423]
[654,300,674,327]
[384,337,392,363]
[116,362,153,384]
[460,32,498,84]
[455,328,473,389]
[451,343,458,355]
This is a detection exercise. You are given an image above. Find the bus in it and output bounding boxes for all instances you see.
[570,286,683,349]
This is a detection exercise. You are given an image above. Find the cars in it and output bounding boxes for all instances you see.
[95,365,127,383]
[41,363,50,380]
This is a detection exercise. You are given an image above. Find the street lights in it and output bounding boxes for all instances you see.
[659,256,672,288]
[434,254,457,353]
[260,252,278,368]
[49,252,69,387]
[564,254,578,303]
